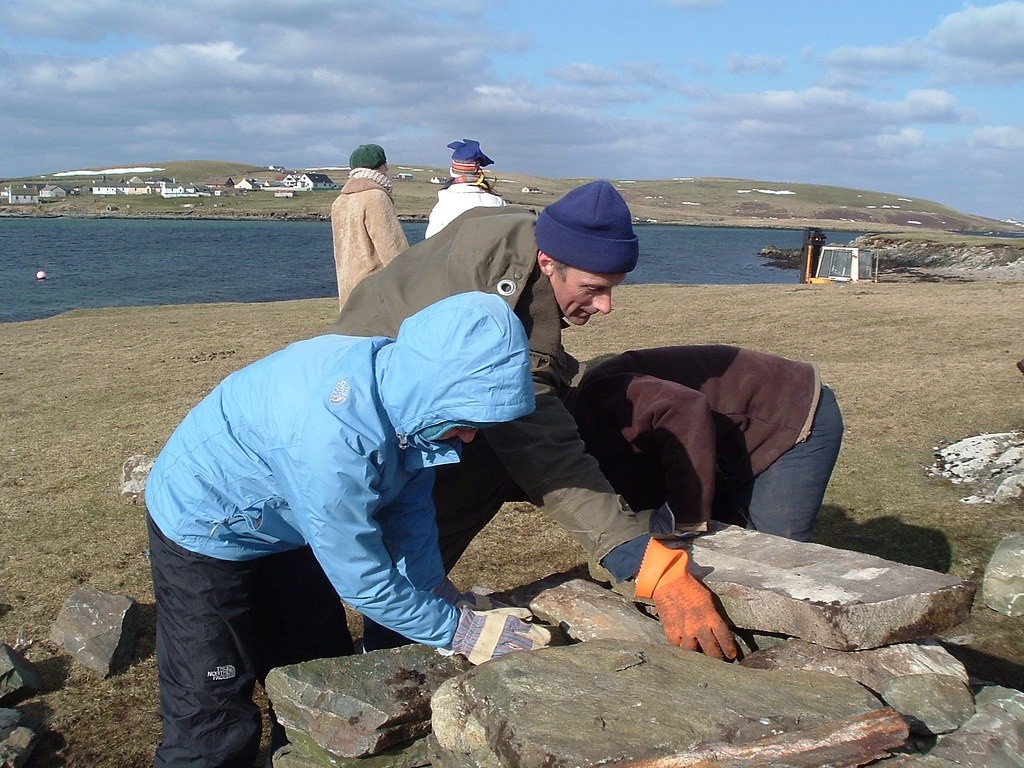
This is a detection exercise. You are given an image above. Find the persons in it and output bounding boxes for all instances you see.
[330,143,411,314]
[320,180,744,663]
[422,140,506,240]
[557,345,844,542]
[144,290,552,768]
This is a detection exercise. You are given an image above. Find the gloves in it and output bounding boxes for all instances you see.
[451,592,551,666]
[635,536,737,661]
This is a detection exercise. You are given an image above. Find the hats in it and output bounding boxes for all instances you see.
[534,180,639,274]
[447,138,495,177]
[349,143,386,169]
